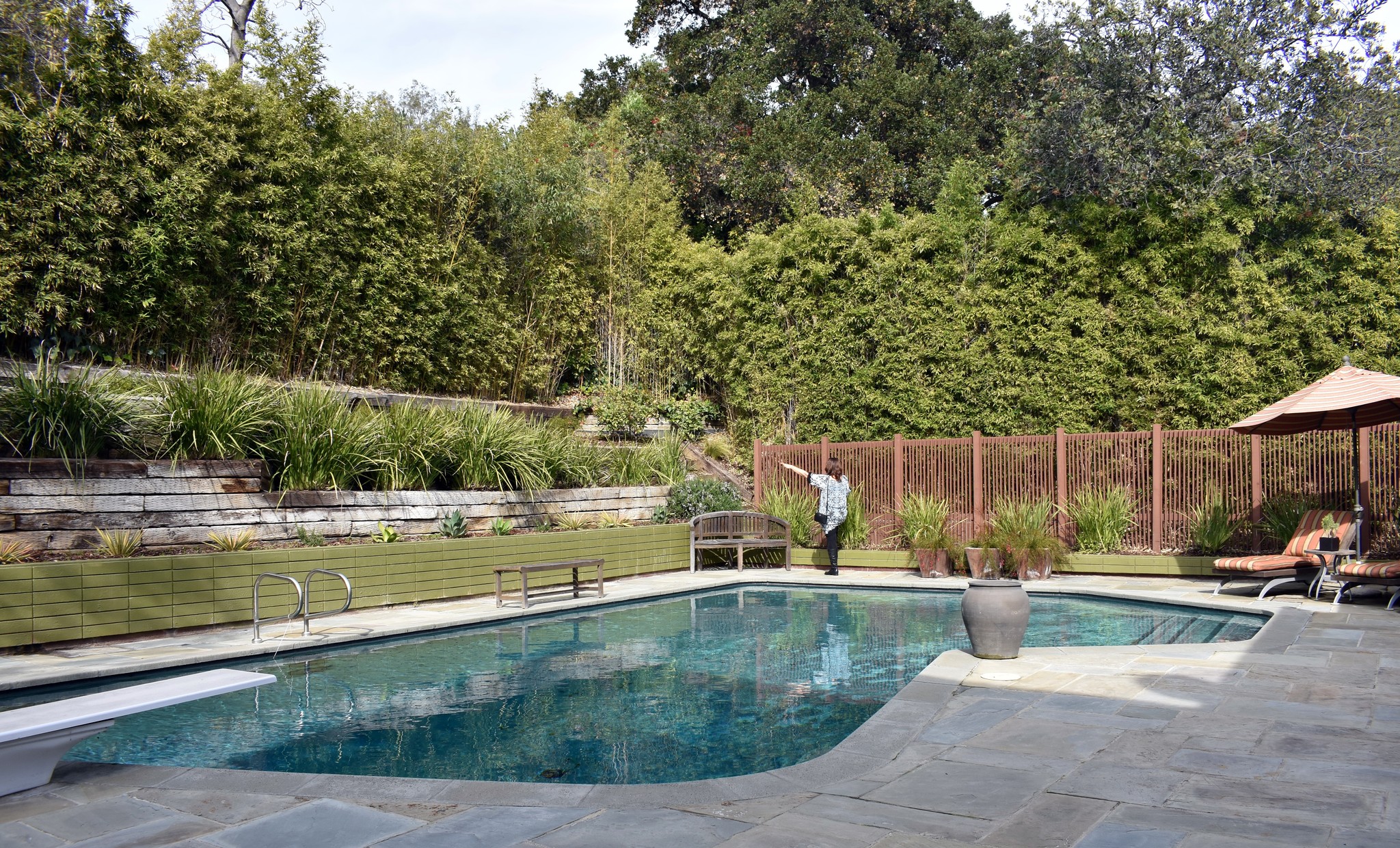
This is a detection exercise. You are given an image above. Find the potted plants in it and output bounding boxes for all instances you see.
[887,484,1073,582]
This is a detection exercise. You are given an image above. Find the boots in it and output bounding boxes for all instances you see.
[824,548,839,576]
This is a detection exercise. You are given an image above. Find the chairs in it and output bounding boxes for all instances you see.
[1318,560,1400,611]
[1212,509,1360,602]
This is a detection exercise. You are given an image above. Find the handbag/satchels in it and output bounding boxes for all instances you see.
[814,511,827,524]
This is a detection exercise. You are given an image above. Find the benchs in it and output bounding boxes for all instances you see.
[688,510,793,574]
[493,557,606,610]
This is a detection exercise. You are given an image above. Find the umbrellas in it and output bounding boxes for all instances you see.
[1228,358,1400,563]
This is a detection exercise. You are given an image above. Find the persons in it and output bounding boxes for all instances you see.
[781,457,848,576]
